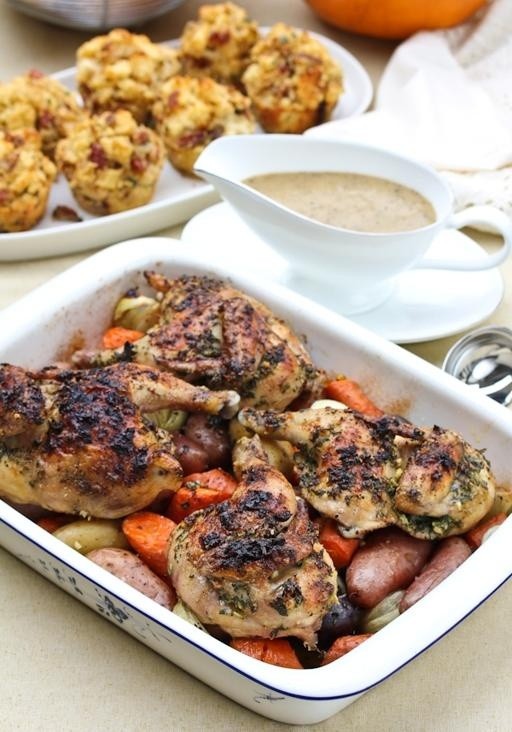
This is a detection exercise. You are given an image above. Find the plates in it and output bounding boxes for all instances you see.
[0,23,377,265]
[0,236,511,728]
[181,201,507,345]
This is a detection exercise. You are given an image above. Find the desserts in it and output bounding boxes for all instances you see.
[0,2,340,234]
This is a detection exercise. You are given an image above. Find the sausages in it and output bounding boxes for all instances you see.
[37,290,505,669]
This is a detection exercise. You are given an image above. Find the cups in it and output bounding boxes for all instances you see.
[190,133,512,317]
